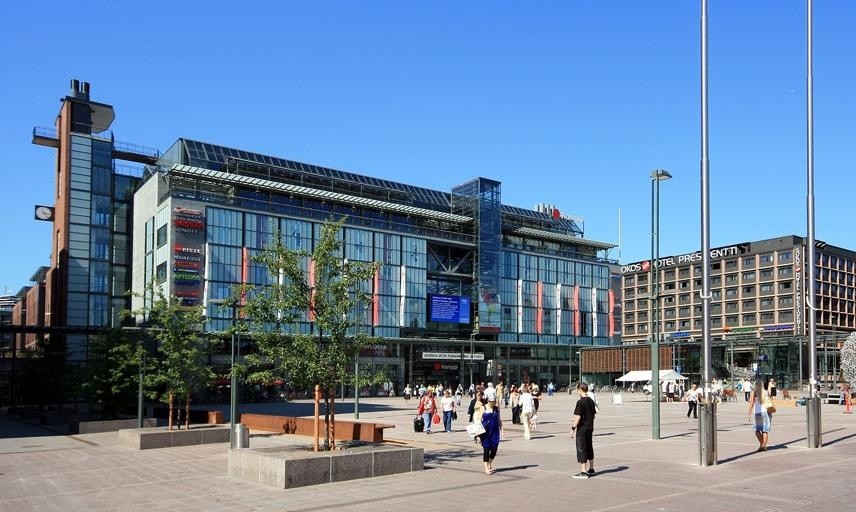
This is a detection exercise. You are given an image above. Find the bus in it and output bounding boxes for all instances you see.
[202,376,294,403]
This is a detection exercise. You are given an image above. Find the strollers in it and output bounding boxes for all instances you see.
[722,378,735,396]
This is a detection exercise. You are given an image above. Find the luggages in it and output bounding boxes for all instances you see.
[414,414,424,432]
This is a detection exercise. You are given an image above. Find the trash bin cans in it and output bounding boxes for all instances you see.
[796,398,806,406]
[234,423,249,448]
[567,387,571,394]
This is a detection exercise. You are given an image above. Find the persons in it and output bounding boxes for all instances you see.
[659,377,775,419]
[403,382,602,479]
[747,380,773,450]
[631,382,636,393]
[260,389,268,402]
[480,286,499,323]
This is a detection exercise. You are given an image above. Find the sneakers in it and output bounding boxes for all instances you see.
[573,469,595,478]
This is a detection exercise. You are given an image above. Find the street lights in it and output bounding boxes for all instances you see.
[830,314,837,374]
[757,326,765,337]
[647,166,674,442]
[575,351,580,383]
[208,297,269,449]
[468,330,480,385]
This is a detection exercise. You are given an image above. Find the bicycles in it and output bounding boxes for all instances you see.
[601,384,618,392]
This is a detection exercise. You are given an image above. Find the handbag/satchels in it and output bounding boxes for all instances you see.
[767,407,776,414]
[433,415,440,423]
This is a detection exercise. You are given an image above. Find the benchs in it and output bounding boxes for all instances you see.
[153,407,224,424]
[771,399,799,407]
[236,411,395,443]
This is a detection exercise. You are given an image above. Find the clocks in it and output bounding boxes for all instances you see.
[35,206,51,220]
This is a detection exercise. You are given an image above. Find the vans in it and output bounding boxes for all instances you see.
[643,379,685,396]
